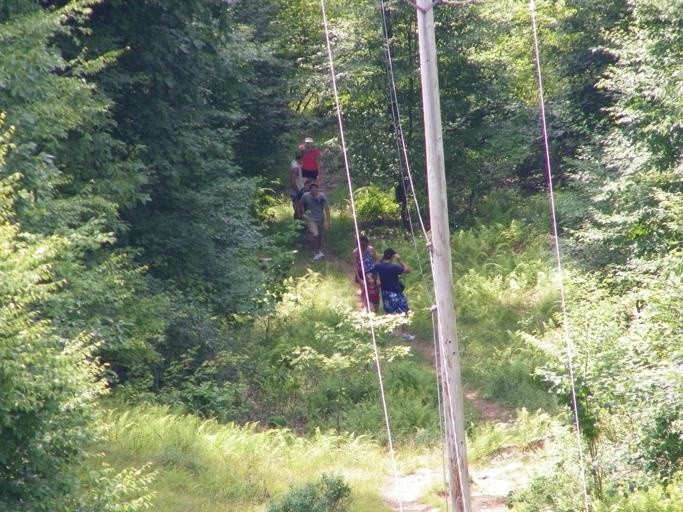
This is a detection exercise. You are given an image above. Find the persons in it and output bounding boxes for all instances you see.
[353,235,381,290]
[289,136,333,260]
[362,273,380,313]
[375,248,416,341]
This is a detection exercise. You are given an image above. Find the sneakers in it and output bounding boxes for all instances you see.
[313,252,324,260]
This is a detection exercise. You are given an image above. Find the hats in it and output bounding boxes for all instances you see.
[384,248,395,254]
